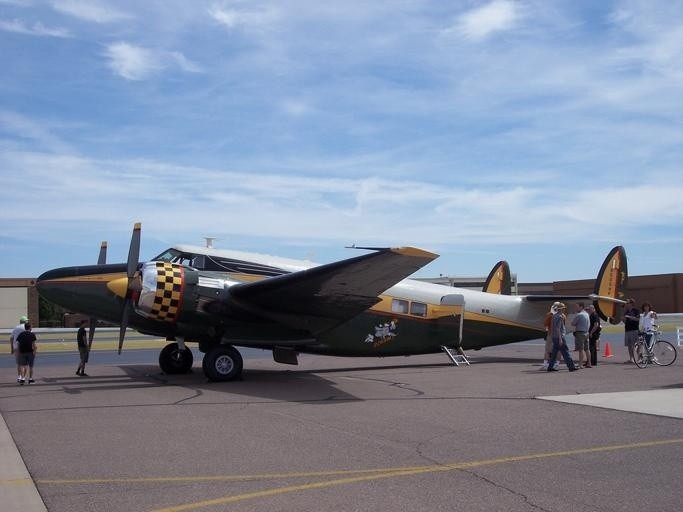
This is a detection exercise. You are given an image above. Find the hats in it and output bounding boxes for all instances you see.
[20,317,29,321]
[557,303,566,310]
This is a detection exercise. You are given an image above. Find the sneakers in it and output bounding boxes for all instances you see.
[76,371,87,375]
[569,365,592,371]
[17,377,35,385]
[539,365,559,372]
[624,359,644,365]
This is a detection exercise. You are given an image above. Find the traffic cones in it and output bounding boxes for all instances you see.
[602,342,612,358]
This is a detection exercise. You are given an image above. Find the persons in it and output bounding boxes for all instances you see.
[76,319,90,376]
[16,323,37,386]
[9,316,29,383]
[621,296,640,363]
[640,301,657,363]
[541,300,602,372]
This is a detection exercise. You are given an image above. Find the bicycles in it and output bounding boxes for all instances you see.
[633,324,677,370]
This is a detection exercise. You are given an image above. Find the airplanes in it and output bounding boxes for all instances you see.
[35,221,632,384]
